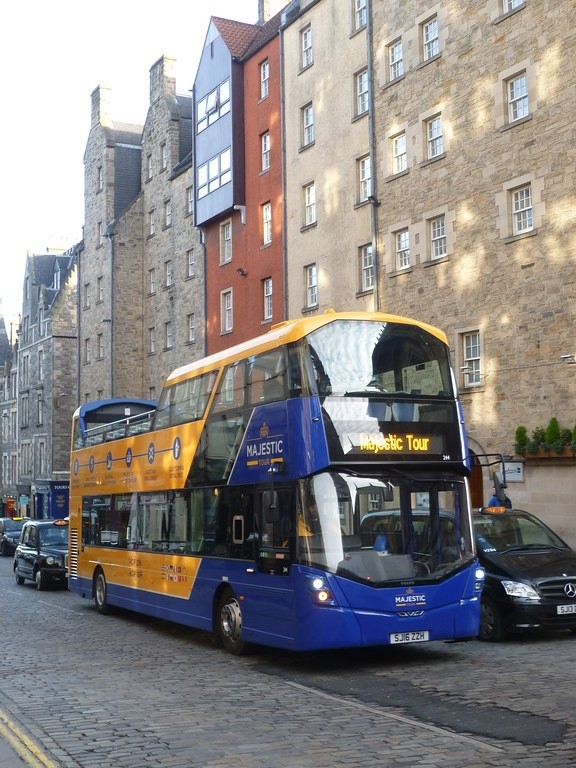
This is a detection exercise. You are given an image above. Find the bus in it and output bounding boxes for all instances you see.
[66,307,514,658]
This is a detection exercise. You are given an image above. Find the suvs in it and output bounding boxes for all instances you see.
[0,517,32,557]
[357,504,576,643]
[12,518,69,591]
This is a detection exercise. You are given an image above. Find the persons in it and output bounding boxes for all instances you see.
[307,495,334,541]
[488,488,515,543]
[488,521,514,549]
[269,380,283,399]
